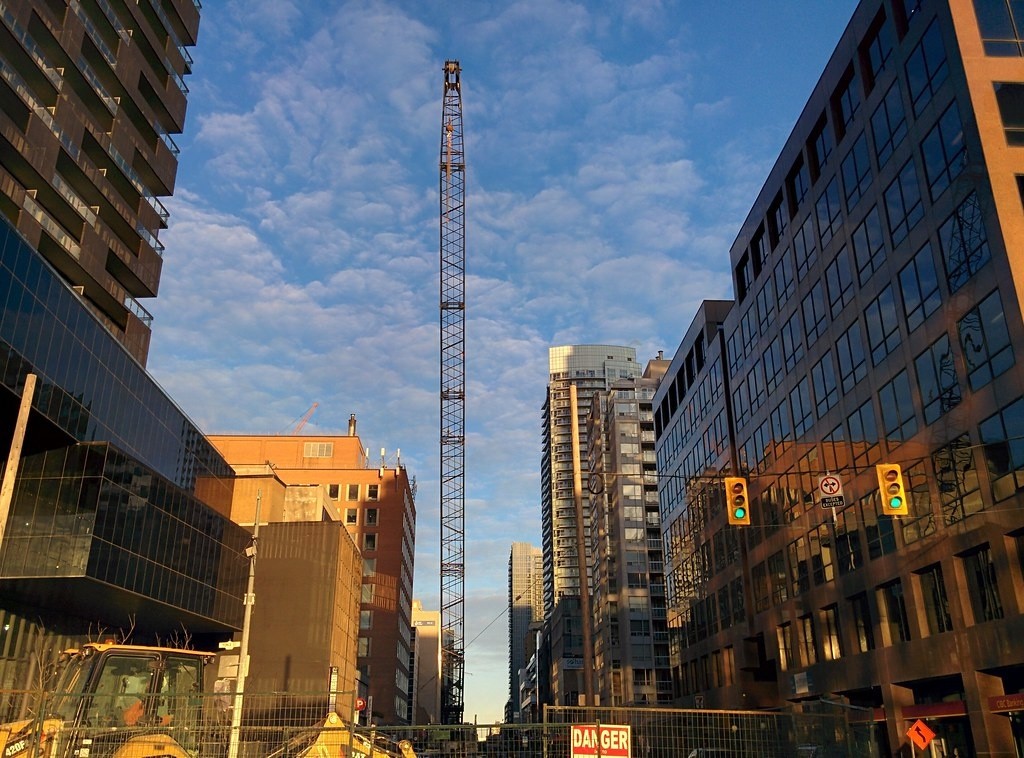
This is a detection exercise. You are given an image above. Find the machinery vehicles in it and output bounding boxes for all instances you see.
[0,639,420,758]
[423,714,488,758]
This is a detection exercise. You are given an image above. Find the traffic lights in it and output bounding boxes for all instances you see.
[877,464,908,515]
[724,478,750,525]
